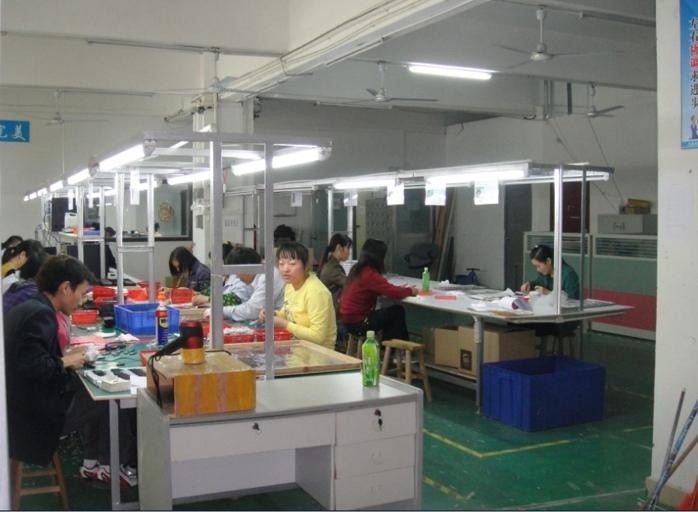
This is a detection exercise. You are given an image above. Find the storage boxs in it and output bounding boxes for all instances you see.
[597,214,658,236]
[480,354,606,432]
[434,325,458,369]
[457,321,536,377]
[69,279,290,354]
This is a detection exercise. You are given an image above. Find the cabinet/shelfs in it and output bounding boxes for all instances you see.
[135,369,424,511]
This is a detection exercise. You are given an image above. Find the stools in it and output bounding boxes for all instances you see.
[10,452,70,510]
[344,333,367,359]
[542,333,576,352]
[381,338,433,402]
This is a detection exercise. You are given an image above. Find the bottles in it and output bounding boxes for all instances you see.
[421,266,430,293]
[154,300,170,348]
[361,330,381,388]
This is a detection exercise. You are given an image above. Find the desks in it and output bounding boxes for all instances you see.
[61,270,300,510]
[344,258,636,418]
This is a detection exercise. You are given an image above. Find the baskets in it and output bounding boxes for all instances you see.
[71,309,99,325]
[112,303,178,336]
[201,322,293,342]
[92,280,160,308]
[166,287,193,304]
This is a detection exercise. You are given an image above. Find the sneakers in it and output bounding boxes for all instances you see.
[78,461,138,490]
[386,354,418,371]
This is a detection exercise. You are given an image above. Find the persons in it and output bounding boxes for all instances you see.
[339,239,417,374]
[105,227,116,239]
[154,224,164,236]
[521,243,579,335]
[265,244,336,350]
[168,248,209,293]
[5,255,137,489]
[1,235,69,352]
[273,224,296,268]
[317,233,352,320]
[203,247,286,324]
[192,243,254,309]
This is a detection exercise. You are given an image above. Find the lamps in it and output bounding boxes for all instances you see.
[166,170,213,188]
[99,142,156,173]
[332,170,527,191]
[67,165,97,187]
[405,62,491,83]
[231,145,331,179]
[24,180,66,202]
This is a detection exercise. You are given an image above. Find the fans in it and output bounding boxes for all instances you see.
[24,92,110,132]
[497,7,611,75]
[551,86,621,123]
[342,65,439,105]
[155,51,282,102]
